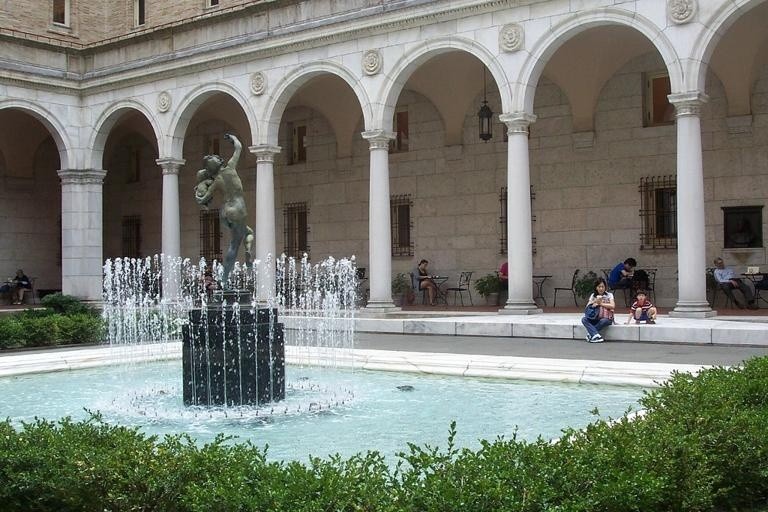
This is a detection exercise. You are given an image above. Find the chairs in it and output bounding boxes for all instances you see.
[497,272,507,305]
[22,277,38,305]
[554,269,579,307]
[600,269,626,307]
[445,271,475,307]
[706,267,735,309]
[410,272,427,305]
[630,269,657,310]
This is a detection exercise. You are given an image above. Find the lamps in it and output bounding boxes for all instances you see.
[477,64,494,143]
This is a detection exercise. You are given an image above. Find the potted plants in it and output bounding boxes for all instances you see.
[475,271,507,305]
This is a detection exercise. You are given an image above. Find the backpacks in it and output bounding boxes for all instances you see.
[630,270,649,289]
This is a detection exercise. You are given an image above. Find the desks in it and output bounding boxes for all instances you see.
[532,276,552,307]
[4,282,26,305]
[627,275,655,283]
[424,276,448,306]
[740,273,768,309]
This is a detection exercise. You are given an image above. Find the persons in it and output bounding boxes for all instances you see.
[623,289,658,325]
[413,260,439,305]
[193,132,255,294]
[581,277,615,343]
[713,256,760,311]
[199,261,213,294]
[607,257,637,290]
[499,262,508,290]
[194,168,214,211]
[12,268,31,305]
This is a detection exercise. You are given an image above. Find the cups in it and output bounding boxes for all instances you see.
[596,295,602,304]
[748,268,753,273]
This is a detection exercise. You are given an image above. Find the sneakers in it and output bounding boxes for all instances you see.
[586,334,591,342]
[647,320,655,324]
[589,337,604,343]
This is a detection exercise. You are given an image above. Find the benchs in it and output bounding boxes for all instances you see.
[37,289,62,299]
[213,289,253,302]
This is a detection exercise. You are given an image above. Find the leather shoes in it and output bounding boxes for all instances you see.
[746,302,759,310]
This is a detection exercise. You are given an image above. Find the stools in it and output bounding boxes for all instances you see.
[365,289,370,307]
